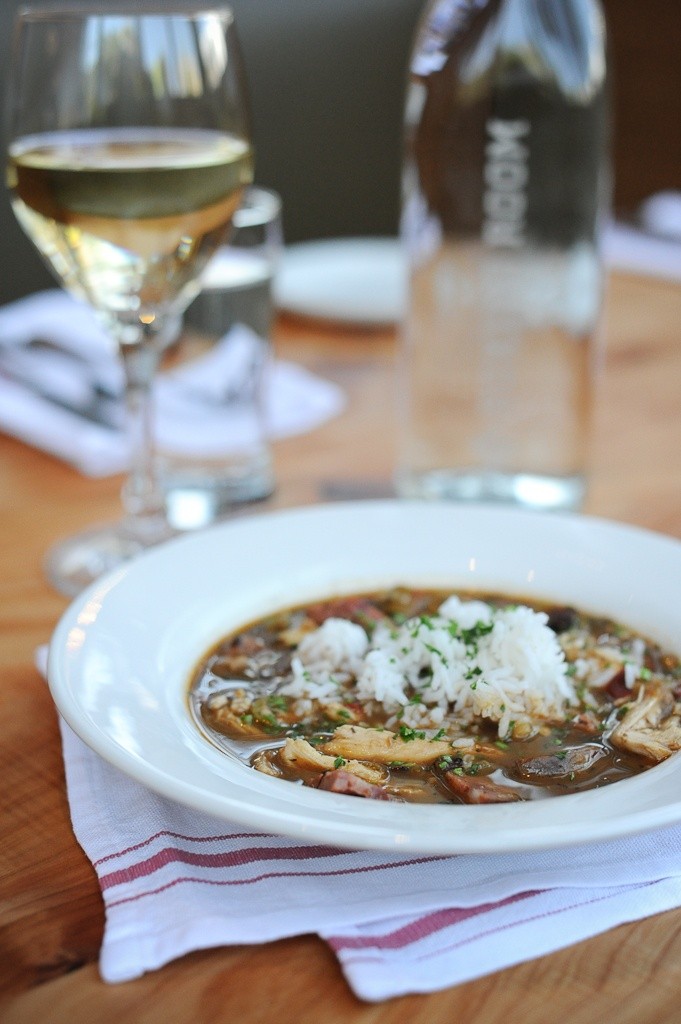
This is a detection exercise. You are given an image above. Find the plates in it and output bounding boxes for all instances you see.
[45,501,681,855]
[280,239,609,338]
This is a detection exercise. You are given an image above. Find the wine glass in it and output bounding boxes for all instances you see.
[3,3,254,603]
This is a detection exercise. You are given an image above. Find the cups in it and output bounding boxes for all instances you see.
[145,200,285,503]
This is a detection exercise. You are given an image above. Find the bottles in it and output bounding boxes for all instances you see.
[386,3,606,510]
